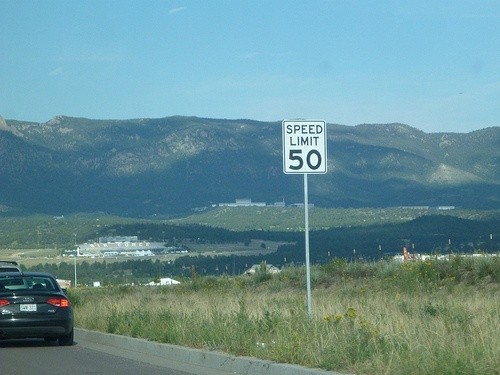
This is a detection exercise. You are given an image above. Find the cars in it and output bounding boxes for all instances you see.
[0,259,29,290]
[0,270,77,347]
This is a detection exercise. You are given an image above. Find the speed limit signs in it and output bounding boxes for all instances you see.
[283,119,328,175]
